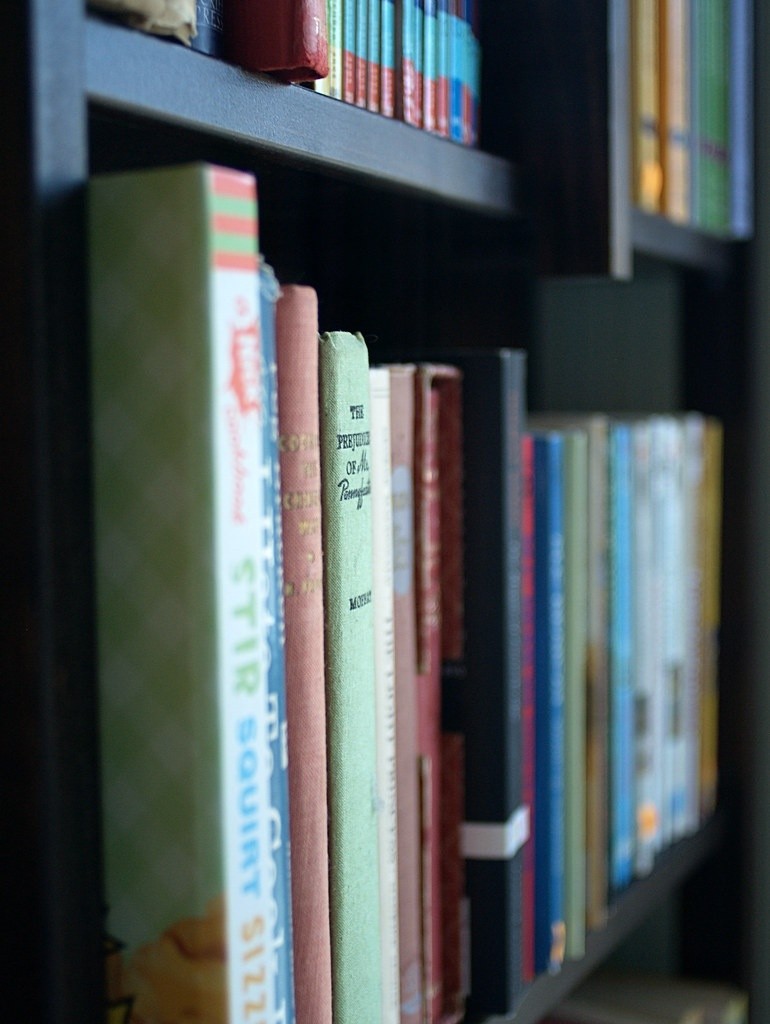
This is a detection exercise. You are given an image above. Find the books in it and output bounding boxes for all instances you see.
[628,0,756,241]
[88,159,750,1024]
[89,0,481,149]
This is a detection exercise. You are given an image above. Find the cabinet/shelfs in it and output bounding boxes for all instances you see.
[0,0,770,1024]
